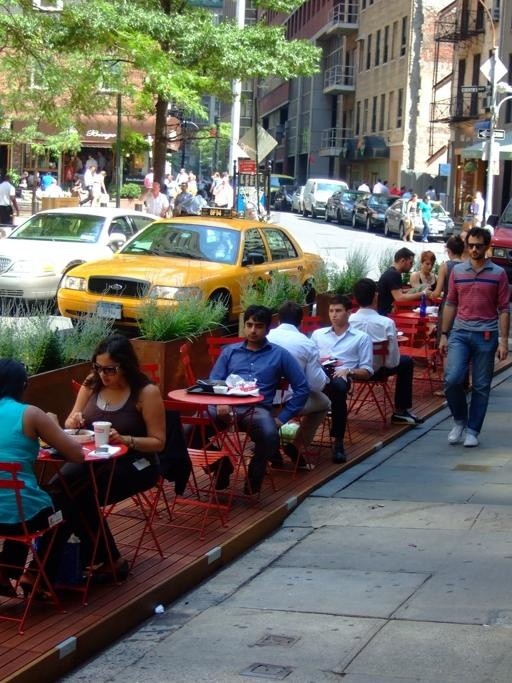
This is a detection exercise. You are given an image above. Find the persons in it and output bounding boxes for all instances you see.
[18,171,28,189]
[172,182,193,214]
[188,170,197,183]
[0,174,20,224]
[40,172,58,190]
[78,165,96,206]
[1,359,85,598]
[402,193,418,242]
[166,174,180,211]
[418,194,433,243]
[472,192,485,226]
[402,188,414,198]
[425,186,436,201]
[210,172,220,193]
[70,176,93,204]
[411,252,438,292]
[383,180,389,187]
[28,171,41,188]
[357,180,371,193]
[309,294,373,463]
[377,247,432,316]
[439,227,511,447]
[176,168,189,182]
[265,304,332,466]
[372,179,384,194]
[348,278,424,426]
[143,167,154,192]
[91,170,109,206]
[464,194,479,222]
[84,154,98,171]
[399,184,406,196]
[186,304,310,496]
[389,183,401,196]
[212,177,234,209]
[178,190,209,216]
[428,237,464,397]
[66,335,166,586]
[145,180,169,217]
[97,152,107,169]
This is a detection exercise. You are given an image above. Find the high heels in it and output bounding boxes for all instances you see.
[19,577,51,603]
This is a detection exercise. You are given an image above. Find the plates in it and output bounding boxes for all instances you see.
[62,429,95,443]
[412,309,432,314]
[185,382,267,396]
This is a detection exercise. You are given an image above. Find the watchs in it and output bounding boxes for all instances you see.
[348,368,354,376]
[128,436,136,449]
[440,332,450,337]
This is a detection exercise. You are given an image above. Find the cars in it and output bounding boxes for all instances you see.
[58,217,328,332]
[487,198,512,273]
[385,199,454,242]
[352,194,400,231]
[274,186,300,210]
[325,191,369,225]
[0,206,165,298]
[291,186,305,213]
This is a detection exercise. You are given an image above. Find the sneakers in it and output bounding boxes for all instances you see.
[391,413,425,425]
[447,424,465,445]
[464,432,479,448]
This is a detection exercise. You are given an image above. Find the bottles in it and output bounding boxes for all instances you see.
[420,294,426,317]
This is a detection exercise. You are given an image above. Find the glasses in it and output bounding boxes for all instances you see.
[92,362,120,377]
[467,242,486,249]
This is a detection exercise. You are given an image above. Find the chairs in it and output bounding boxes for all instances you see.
[347,341,395,424]
[266,417,320,481]
[1,462,66,634]
[399,318,442,399]
[205,379,290,497]
[105,475,168,572]
[145,400,231,540]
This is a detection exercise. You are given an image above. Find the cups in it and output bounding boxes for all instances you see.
[93,422,112,447]
[212,386,229,394]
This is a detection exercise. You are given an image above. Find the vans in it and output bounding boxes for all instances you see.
[265,174,296,204]
[303,179,349,218]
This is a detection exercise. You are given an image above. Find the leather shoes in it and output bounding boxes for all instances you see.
[92,558,130,586]
[333,444,347,464]
[214,456,234,491]
[283,442,307,468]
[244,470,264,495]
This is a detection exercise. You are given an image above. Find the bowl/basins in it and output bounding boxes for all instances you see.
[427,306,439,313]
[196,379,226,390]
